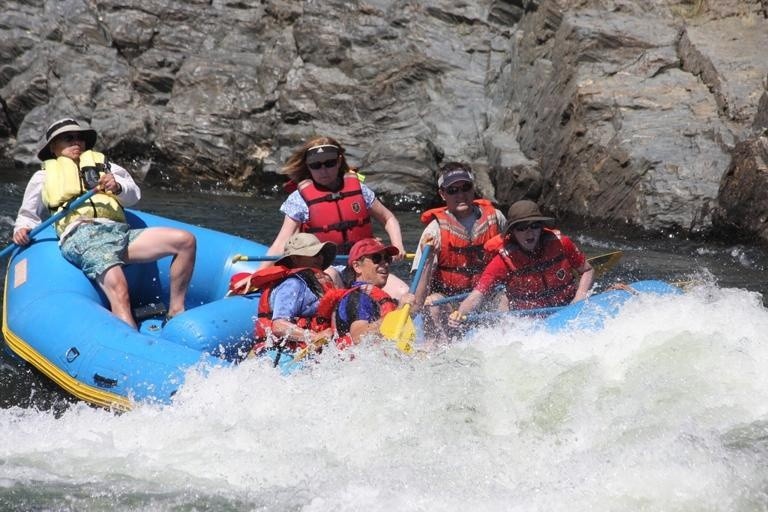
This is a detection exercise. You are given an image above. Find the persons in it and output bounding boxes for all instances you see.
[12,112,199,329]
[408,162,510,343]
[332,231,443,343]
[232,134,411,310]
[251,231,339,359]
[444,197,594,330]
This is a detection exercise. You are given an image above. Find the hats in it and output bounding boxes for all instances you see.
[438,168,473,188]
[504,200,554,236]
[274,233,336,271]
[38,119,97,161]
[348,239,399,266]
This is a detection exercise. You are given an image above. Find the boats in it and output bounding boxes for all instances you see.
[3,205,686,426]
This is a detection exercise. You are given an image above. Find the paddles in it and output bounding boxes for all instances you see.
[378,246,433,361]
[432,250,632,308]
[292,332,330,362]
[229,250,419,263]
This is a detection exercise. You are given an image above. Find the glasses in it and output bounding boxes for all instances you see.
[517,222,540,231]
[309,159,337,169]
[372,253,391,264]
[64,134,86,142]
[447,183,470,194]
